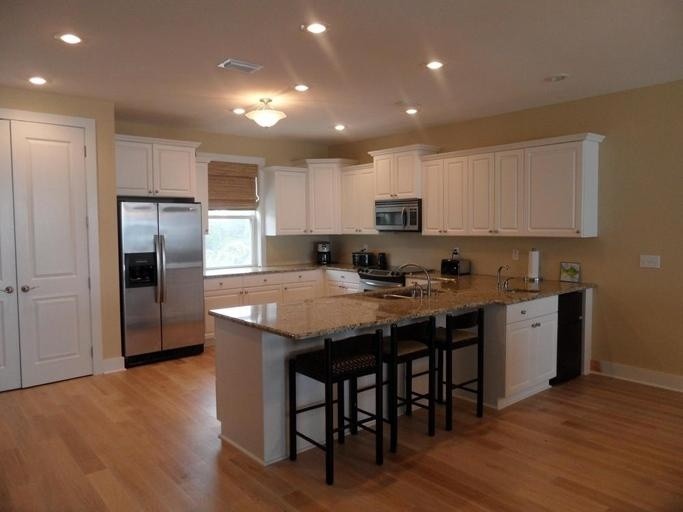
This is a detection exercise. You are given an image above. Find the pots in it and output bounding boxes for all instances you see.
[351,252,373,267]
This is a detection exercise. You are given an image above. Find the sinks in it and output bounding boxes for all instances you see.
[367,293,406,300]
[506,288,541,294]
[391,288,428,297]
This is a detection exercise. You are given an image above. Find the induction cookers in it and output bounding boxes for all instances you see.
[358,265,433,281]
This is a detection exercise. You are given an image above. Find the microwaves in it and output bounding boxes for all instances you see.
[374,200,420,230]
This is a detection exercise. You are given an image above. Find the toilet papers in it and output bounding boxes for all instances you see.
[526,250,540,279]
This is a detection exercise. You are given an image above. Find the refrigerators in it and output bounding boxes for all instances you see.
[117,199,206,364]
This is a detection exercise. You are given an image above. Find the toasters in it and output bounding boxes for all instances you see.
[440,258,470,274]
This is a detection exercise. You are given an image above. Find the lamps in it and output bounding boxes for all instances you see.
[244,95,289,133]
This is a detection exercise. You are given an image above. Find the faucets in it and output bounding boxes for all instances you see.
[397,261,433,297]
[495,264,511,284]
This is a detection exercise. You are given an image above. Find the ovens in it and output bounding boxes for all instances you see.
[359,278,403,293]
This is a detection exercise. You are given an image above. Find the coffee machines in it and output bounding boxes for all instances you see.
[316,241,330,264]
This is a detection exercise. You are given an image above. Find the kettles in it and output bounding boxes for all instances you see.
[377,253,386,267]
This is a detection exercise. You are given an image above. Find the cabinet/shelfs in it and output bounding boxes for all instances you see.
[263,168,306,238]
[421,132,606,241]
[202,265,440,338]
[114,133,201,198]
[503,294,558,399]
[374,150,439,201]
[305,162,343,235]
[341,164,382,235]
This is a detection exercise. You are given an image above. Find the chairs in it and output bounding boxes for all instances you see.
[403,306,486,433]
[287,327,384,486]
[349,313,437,456]
